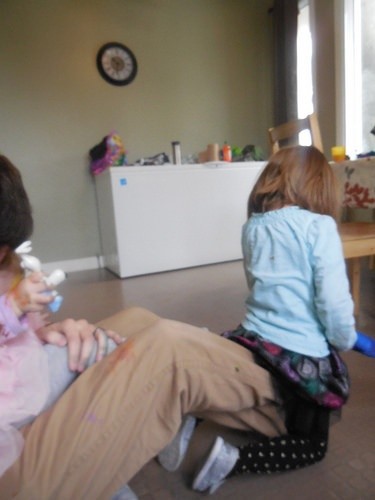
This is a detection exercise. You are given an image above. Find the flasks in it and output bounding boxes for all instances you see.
[172,142,181,164]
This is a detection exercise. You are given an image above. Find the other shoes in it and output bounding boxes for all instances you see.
[156,411,195,471]
[192,437,241,494]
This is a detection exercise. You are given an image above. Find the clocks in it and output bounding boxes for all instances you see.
[96,42,138,87]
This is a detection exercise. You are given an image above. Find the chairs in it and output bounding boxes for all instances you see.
[267,114,375,312]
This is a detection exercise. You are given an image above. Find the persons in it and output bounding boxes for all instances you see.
[160,146,358,493]
[0,157,294,500]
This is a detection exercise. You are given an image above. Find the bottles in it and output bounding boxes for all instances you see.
[222,141,232,162]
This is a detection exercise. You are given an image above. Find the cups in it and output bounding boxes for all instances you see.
[208,143,219,161]
[331,145,345,161]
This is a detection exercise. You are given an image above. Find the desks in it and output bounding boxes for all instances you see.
[95,161,271,279]
